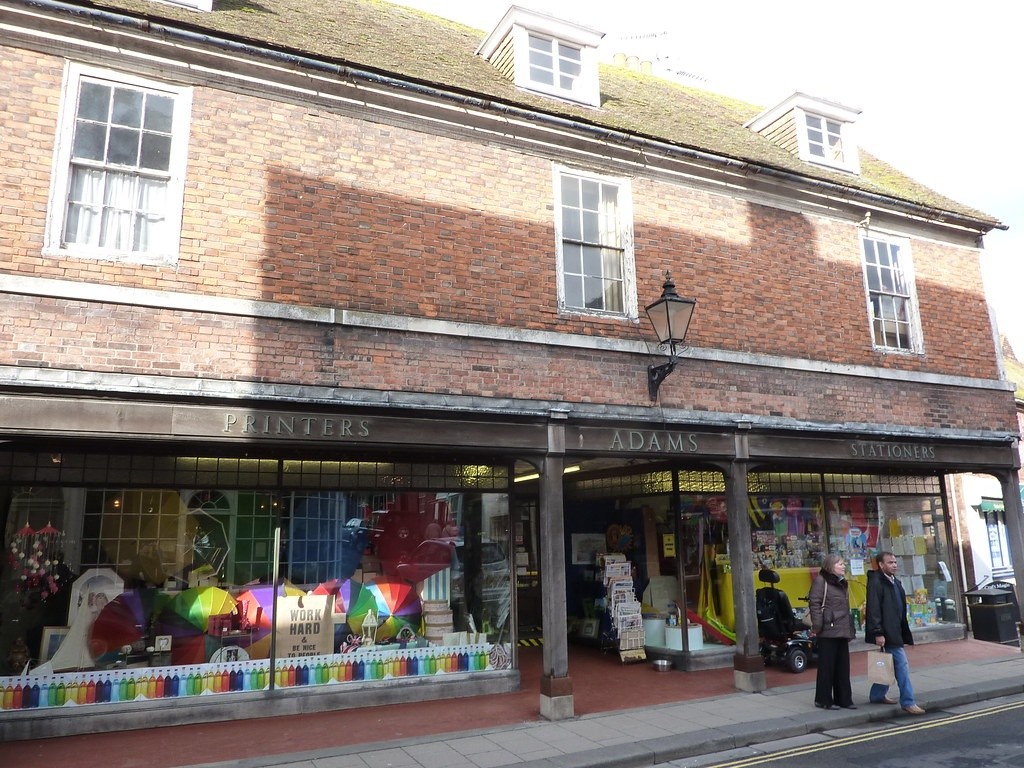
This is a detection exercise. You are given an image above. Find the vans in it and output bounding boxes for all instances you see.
[394,536,514,615]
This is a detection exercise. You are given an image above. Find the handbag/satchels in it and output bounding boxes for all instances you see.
[802,579,827,627]
[867,645,895,686]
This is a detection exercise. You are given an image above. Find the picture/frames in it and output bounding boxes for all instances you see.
[579,618,600,639]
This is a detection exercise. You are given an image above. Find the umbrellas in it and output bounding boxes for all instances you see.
[87,588,171,669]
[346,576,421,645]
[160,576,362,664]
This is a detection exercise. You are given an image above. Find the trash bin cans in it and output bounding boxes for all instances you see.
[963,587,1019,646]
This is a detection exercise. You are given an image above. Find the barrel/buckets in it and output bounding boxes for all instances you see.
[424,610,453,642]
[424,600,448,611]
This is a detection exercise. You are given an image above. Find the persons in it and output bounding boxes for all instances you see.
[809,552,857,710]
[864,552,926,714]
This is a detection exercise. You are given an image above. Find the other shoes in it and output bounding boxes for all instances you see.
[815,702,840,711]
[844,704,857,710]
[903,704,925,715]
[871,695,898,705]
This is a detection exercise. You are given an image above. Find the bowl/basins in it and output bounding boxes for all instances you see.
[653,660,671,672]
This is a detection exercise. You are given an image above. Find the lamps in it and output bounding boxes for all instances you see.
[643,270,696,401]
